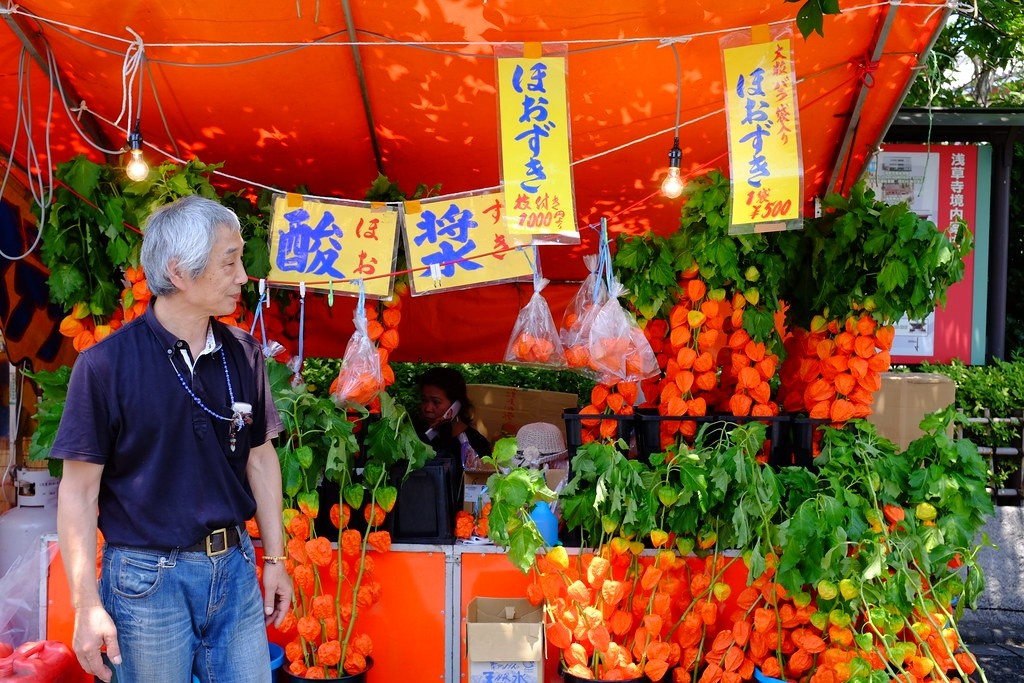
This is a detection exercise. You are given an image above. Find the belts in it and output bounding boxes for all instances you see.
[166,521,246,557]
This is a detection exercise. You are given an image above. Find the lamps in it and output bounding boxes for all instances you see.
[662,39,684,200]
[126,50,149,181]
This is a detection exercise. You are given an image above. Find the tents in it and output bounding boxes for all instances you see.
[1,0,961,377]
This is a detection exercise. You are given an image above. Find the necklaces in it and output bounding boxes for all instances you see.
[167,347,244,452]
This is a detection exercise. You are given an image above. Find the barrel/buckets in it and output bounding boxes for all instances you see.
[268,641,285,683]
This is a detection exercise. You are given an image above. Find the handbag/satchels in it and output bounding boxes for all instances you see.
[502,245,569,369]
[560,217,662,387]
[334,278,384,408]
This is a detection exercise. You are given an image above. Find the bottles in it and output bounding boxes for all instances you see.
[0,638,83,683]
[528,500,558,547]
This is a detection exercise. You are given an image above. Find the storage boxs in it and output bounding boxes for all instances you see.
[466,384,579,452]
[867,371,956,469]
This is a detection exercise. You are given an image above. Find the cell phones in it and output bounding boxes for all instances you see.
[426,400,462,441]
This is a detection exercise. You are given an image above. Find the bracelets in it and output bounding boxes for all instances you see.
[262,555,287,564]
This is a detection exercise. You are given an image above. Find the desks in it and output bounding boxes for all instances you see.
[39,534,943,682]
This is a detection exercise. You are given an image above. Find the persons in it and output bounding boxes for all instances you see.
[49,194,294,683]
[409,367,492,512]
